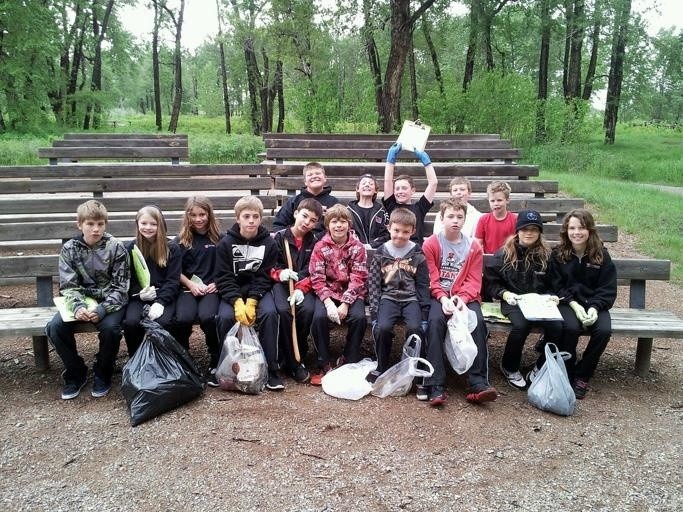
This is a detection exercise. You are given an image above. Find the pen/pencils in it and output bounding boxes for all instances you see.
[132,288,159,297]
[183,291,192,293]
[554,297,565,302]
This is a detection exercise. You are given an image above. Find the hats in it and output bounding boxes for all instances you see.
[515,209,543,234]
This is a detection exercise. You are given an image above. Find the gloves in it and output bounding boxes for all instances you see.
[279,268,299,282]
[140,284,157,302]
[387,142,402,166]
[233,297,258,327]
[569,300,599,327]
[551,295,560,305]
[323,297,341,325]
[287,289,305,306]
[414,147,431,166]
[502,291,521,306]
[147,302,165,320]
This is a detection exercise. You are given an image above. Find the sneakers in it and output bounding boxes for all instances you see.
[429,384,450,407]
[310,354,347,386]
[207,367,221,387]
[284,361,310,383]
[416,383,429,400]
[266,376,284,390]
[572,376,588,399]
[526,363,540,384]
[366,371,382,384]
[464,388,497,404]
[91,372,113,398]
[500,362,528,390]
[61,376,89,400]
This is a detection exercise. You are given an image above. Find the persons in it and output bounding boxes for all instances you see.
[271,198,321,383]
[366,207,430,402]
[207,195,286,391]
[432,176,480,240]
[484,209,555,390]
[46,199,130,400]
[422,198,497,408]
[544,207,618,399]
[272,162,340,245]
[383,141,438,247]
[121,204,180,360]
[344,173,387,246]
[172,195,223,388]
[307,204,366,372]
[472,180,520,304]
